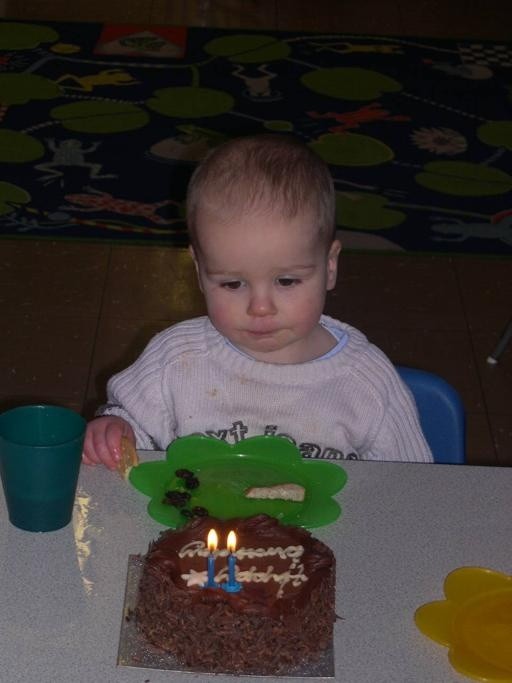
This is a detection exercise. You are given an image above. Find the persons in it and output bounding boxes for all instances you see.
[80,129,435,475]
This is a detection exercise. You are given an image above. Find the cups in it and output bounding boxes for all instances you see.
[0,405,87,532]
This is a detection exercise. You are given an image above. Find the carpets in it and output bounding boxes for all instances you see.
[0,22,512,259]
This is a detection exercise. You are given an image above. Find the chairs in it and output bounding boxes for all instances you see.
[397,366,467,465]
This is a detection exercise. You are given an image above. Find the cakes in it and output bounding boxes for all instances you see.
[135,514,345,676]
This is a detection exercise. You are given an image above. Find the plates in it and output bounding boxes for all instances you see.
[128,434,348,530]
[414,565,512,683]
